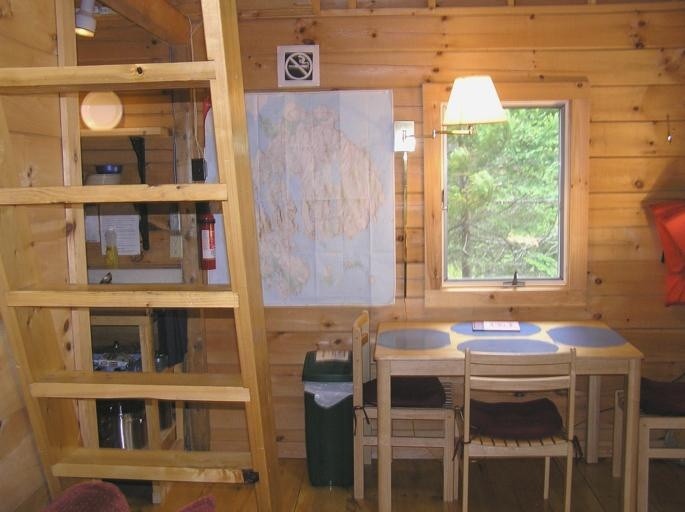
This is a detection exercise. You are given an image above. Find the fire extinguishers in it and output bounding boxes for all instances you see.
[198,207,216,270]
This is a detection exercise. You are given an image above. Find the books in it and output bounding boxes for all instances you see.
[471,321,519,331]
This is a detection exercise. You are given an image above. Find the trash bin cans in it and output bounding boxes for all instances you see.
[301,349,354,487]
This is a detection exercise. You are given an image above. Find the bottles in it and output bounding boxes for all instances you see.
[104,225,119,266]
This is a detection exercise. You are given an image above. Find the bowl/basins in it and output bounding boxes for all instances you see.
[94,162,123,173]
[90,340,170,373]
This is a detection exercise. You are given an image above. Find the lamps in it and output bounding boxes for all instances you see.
[74,0,111,37]
[392,74,510,152]
